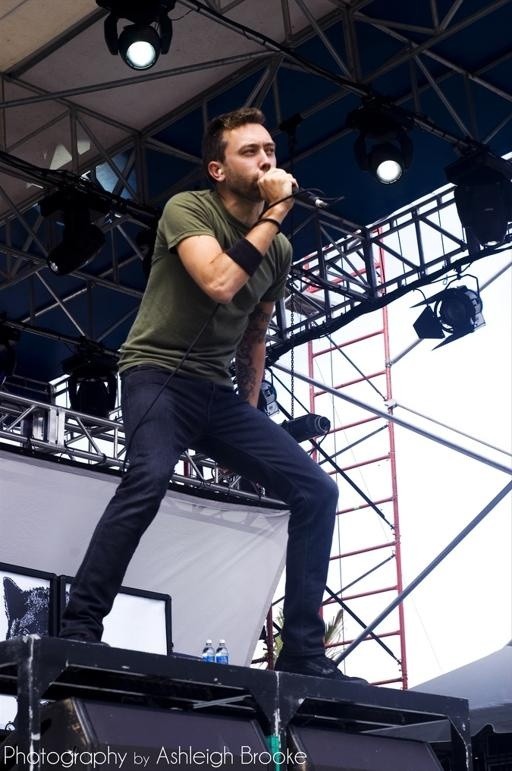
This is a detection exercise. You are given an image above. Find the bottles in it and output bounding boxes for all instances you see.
[214,639,229,665]
[200,639,216,663]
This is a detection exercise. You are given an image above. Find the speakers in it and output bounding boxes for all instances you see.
[287,725,445,771]
[0,697,275,771]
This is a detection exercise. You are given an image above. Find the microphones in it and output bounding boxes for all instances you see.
[293,185,328,209]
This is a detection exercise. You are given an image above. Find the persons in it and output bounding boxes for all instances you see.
[60,106,366,683]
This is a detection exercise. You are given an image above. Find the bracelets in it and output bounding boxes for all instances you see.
[225,241,262,275]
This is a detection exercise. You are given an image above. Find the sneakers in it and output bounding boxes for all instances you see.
[274,650,368,684]
[65,632,110,647]
